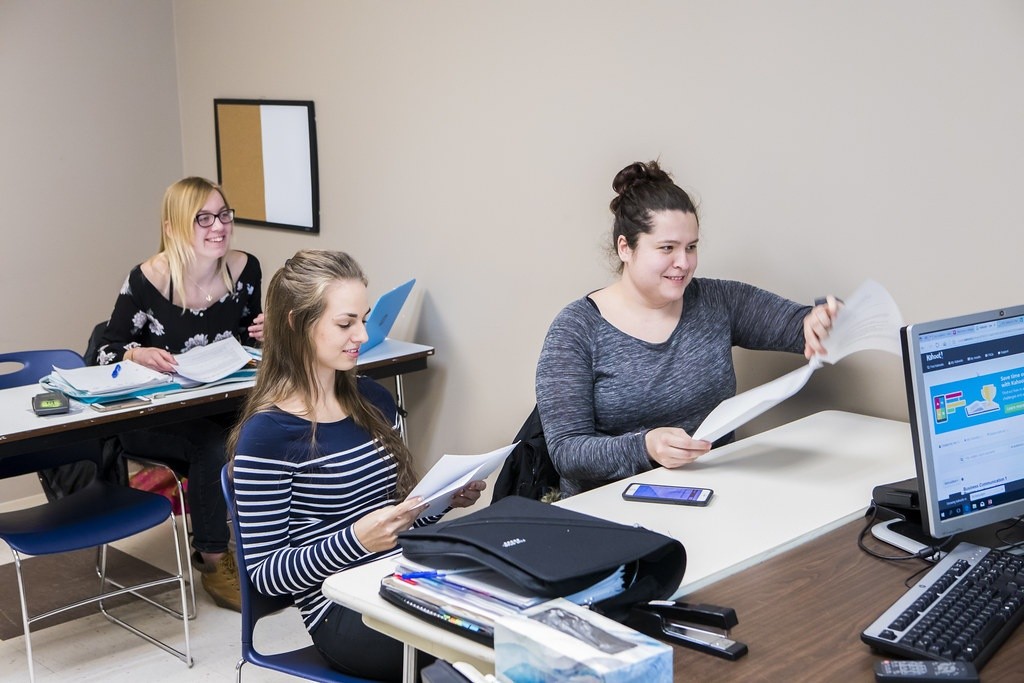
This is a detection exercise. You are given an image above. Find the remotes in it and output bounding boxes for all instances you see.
[874,659,980,683]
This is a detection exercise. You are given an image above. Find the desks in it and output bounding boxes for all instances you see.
[0,336,435,485]
[322,408,1023,682]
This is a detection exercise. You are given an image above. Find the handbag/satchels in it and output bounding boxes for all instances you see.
[379,496,687,653]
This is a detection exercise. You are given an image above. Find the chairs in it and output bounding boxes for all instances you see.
[219,460,367,682]
[1,323,198,682]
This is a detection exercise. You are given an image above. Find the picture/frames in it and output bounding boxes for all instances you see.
[214,100,319,234]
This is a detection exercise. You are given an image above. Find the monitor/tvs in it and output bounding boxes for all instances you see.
[871,303,1024,565]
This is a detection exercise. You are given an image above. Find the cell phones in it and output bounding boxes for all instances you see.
[623,483,714,506]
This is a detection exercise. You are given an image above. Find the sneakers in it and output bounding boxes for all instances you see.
[190,549,242,612]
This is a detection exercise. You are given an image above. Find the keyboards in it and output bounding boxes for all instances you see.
[860,542,1024,670]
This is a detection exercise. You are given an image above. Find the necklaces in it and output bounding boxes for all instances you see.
[185,273,218,302]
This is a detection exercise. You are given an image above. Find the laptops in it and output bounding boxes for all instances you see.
[356,278,416,354]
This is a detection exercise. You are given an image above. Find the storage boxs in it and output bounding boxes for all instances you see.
[493,597,674,682]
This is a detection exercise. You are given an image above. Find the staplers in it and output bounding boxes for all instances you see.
[629,599,748,661]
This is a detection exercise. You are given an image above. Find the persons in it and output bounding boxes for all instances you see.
[535,159,839,501]
[92,177,266,612]
[228,247,487,683]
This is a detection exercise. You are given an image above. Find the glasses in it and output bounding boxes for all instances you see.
[193,209,235,227]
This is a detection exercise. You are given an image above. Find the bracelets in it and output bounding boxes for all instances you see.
[125,348,136,361]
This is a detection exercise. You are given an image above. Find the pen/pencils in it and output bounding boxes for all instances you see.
[401,565,491,580]
[111,364,121,378]
